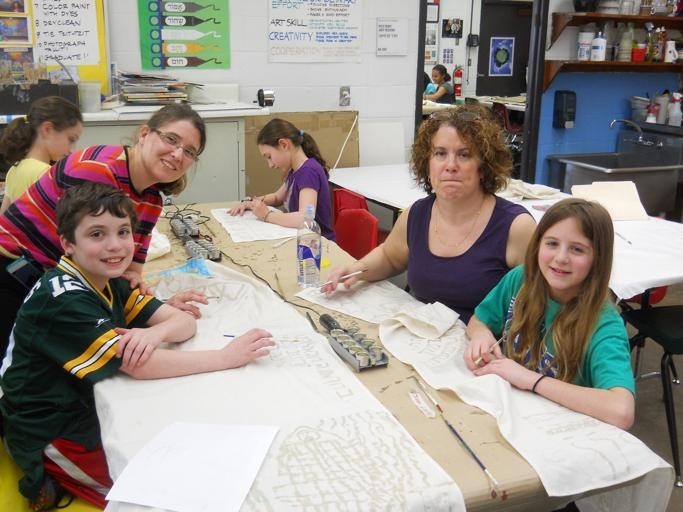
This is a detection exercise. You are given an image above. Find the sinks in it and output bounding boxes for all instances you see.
[545,152,683,215]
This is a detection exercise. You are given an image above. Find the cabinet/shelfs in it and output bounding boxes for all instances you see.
[0,106,269,202]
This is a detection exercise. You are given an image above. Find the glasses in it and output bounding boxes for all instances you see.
[152,128,197,160]
[427,110,480,122]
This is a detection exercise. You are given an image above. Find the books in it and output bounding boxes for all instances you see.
[108,67,199,115]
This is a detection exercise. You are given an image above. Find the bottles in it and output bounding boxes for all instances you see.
[661,26,668,58]
[615,22,626,43]
[603,20,615,45]
[617,31,633,61]
[576,31,594,61]
[584,21,599,32]
[611,43,618,60]
[652,27,662,61]
[110,64,118,94]
[605,42,611,59]
[627,22,633,39]
[666,0,674,16]
[297,204,321,289]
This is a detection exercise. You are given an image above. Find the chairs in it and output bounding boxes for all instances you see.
[332,189,368,226]
[620,290,682,487]
[489,102,524,148]
[333,209,379,260]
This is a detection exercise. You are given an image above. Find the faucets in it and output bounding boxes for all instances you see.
[609,119,644,142]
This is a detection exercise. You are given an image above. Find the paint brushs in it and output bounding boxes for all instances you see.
[223,334,303,343]
[163,296,217,302]
[414,375,443,413]
[614,229,633,246]
[318,268,370,287]
[474,333,507,366]
[440,412,498,484]
[250,196,266,219]
[273,238,294,247]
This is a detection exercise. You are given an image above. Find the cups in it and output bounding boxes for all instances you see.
[632,47,646,63]
[665,41,678,63]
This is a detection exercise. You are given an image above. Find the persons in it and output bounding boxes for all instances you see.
[7,183,279,508]
[316,111,543,327]
[1,105,211,369]
[468,198,646,428]
[3,94,84,212]
[423,64,455,104]
[423,72,434,101]
[228,119,337,243]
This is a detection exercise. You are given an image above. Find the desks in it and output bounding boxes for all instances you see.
[325,164,682,385]
[93,198,646,511]
[421,100,457,114]
[470,89,525,111]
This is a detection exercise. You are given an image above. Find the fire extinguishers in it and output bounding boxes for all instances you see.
[453,64,462,96]
